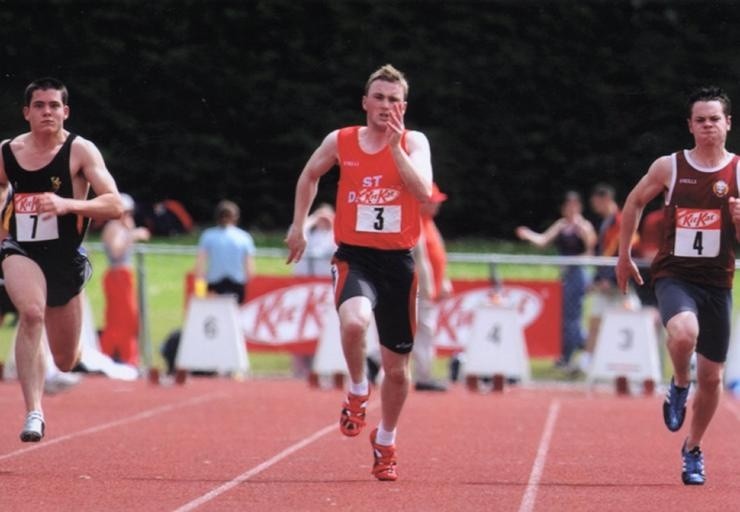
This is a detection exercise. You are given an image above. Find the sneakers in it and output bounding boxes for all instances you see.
[341,384,371,436]
[664,376,690,431]
[21,411,45,442]
[682,440,706,484]
[372,429,398,480]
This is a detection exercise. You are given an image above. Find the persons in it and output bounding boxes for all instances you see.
[1,77,128,444]
[191,196,258,309]
[612,87,740,487]
[286,60,435,485]
[96,190,154,372]
[285,205,340,279]
[561,184,641,377]
[631,196,667,307]
[415,176,455,395]
[515,190,600,372]
